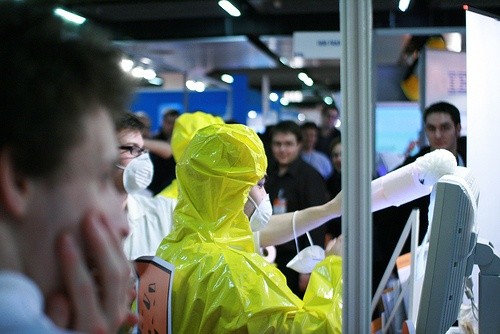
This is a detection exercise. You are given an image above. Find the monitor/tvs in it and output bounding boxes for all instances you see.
[415,166,479,334]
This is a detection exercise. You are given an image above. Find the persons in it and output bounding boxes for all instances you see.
[0,8,132,334]
[114,101,467,320]
[130,124,343,334]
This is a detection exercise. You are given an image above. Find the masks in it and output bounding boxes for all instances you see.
[286,209,324,274]
[246,194,273,232]
[116,153,154,195]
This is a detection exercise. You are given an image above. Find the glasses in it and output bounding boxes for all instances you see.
[115,145,147,156]
